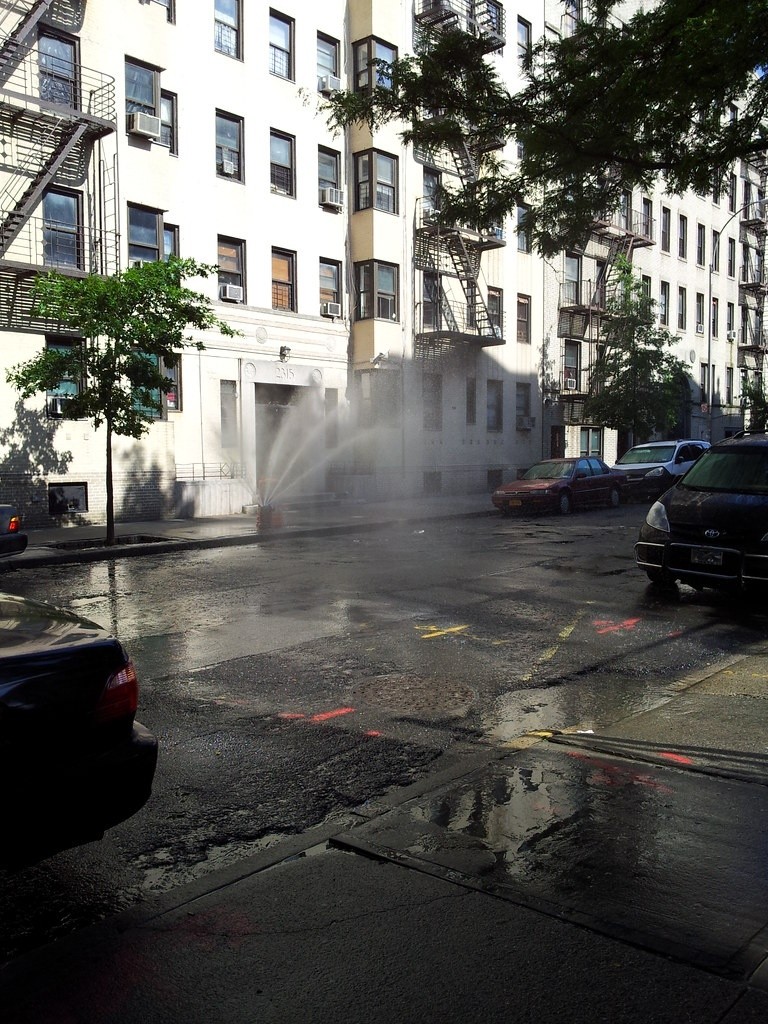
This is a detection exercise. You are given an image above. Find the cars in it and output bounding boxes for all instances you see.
[0,591,159,877]
[492,454,630,515]
[0,503,30,557]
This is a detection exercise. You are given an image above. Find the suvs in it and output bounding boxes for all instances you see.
[612,437,712,498]
[635,428,768,593]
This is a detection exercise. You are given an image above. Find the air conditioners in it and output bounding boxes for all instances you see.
[564,377,577,390]
[321,302,341,317]
[728,330,737,340]
[220,284,243,302]
[319,187,345,208]
[318,75,342,93]
[127,112,162,139]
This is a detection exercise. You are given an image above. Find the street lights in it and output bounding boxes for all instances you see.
[708,198,768,442]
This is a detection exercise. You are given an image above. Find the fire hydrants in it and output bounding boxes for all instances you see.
[256,500,273,531]
